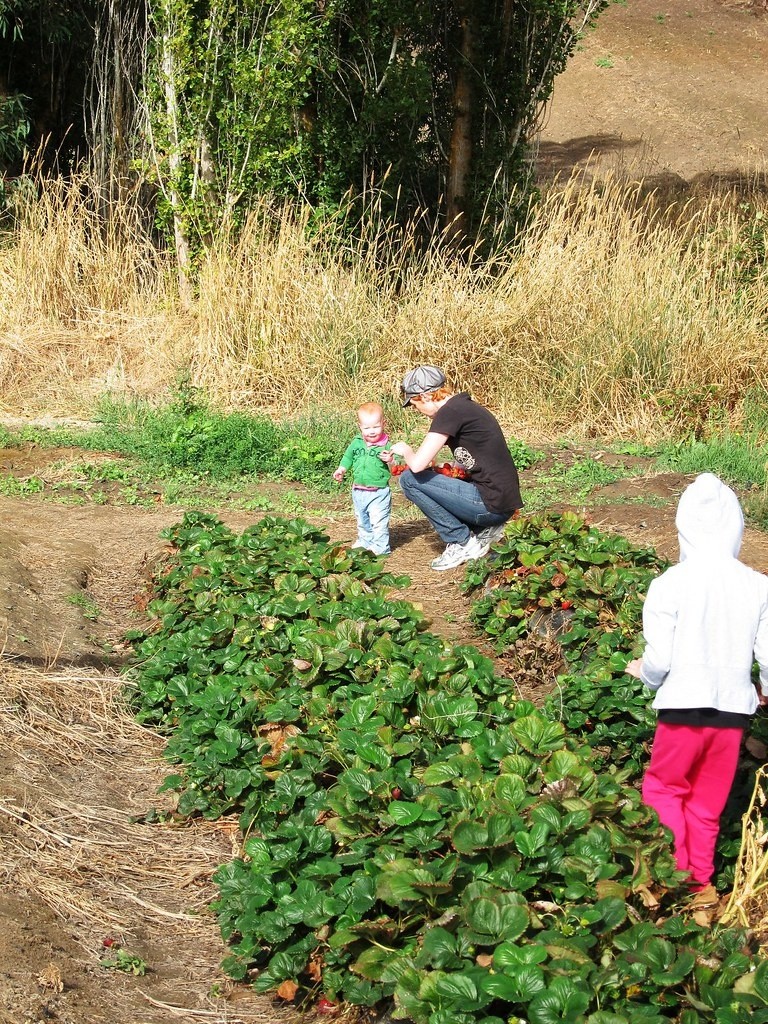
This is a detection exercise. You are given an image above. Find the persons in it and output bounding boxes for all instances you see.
[334,402,402,556]
[388,366,525,571]
[624,472,767,892]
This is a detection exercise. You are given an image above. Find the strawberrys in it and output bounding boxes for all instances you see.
[391,464,408,476]
[393,789,401,799]
[562,602,569,609]
[434,463,465,479]
[320,1000,333,1014]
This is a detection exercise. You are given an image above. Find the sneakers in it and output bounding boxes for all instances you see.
[431,525,507,571]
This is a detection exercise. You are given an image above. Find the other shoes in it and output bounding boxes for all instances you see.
[351,539,390,557]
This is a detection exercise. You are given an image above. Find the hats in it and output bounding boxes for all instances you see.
[402,366,447,408]
